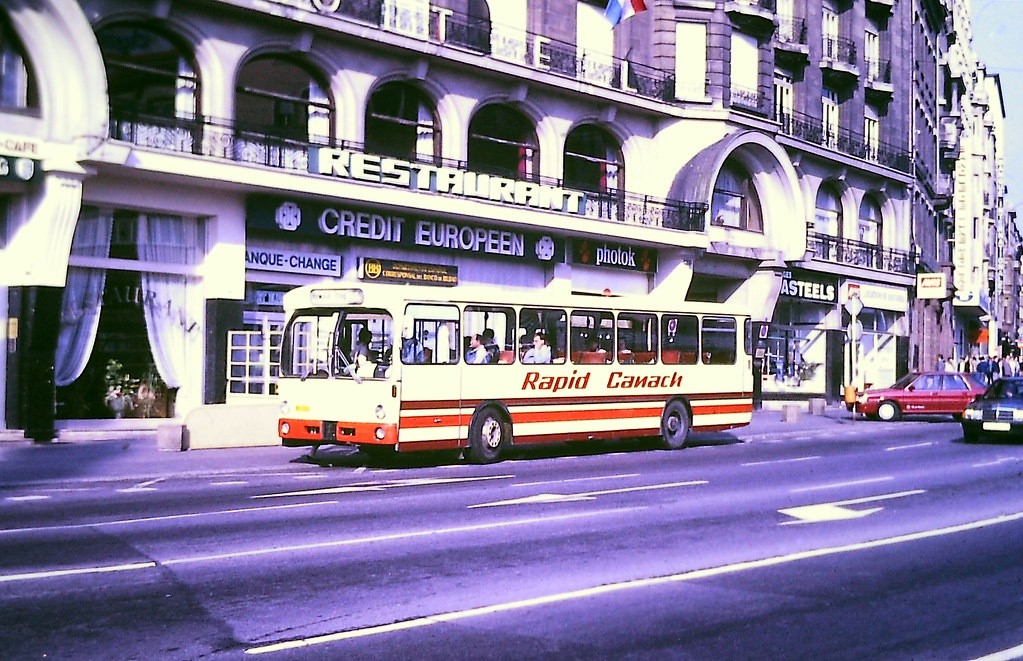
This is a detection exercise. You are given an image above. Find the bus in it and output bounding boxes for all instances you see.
[276,281,760,456]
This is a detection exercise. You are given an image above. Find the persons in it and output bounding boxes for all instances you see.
[616,337,632,354]
[519,334,555,357]
[466,333,490,365]
[936,351,1023,398]
[481,329,500,365]
[524,332,552,364]
[587,336,606,354]
[350,329,372,366]
[384,330,423,366]
[464,336,471,360]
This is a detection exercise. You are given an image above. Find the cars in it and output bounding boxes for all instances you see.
[961,377,1023,444]
[844,371,990,422]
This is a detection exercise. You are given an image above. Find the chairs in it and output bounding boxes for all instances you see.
[352,332,735,378]
